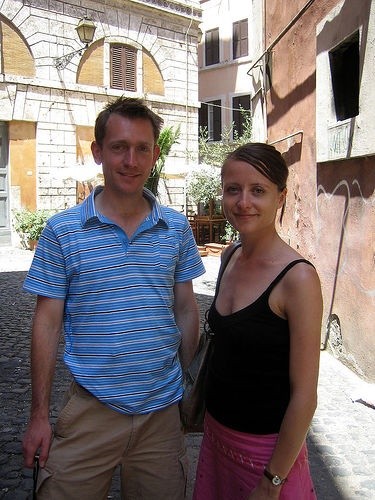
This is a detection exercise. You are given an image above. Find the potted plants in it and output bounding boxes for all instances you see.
[15,206,57,251]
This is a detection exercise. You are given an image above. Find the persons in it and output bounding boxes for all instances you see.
[22,93,206,500]
[192,142,324,500]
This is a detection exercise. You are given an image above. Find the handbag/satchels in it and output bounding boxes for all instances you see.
[180,240,242,435]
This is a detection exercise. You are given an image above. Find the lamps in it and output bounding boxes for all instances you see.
[53,15,97,66]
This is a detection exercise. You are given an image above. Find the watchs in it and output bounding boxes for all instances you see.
[263,469,288,487]
